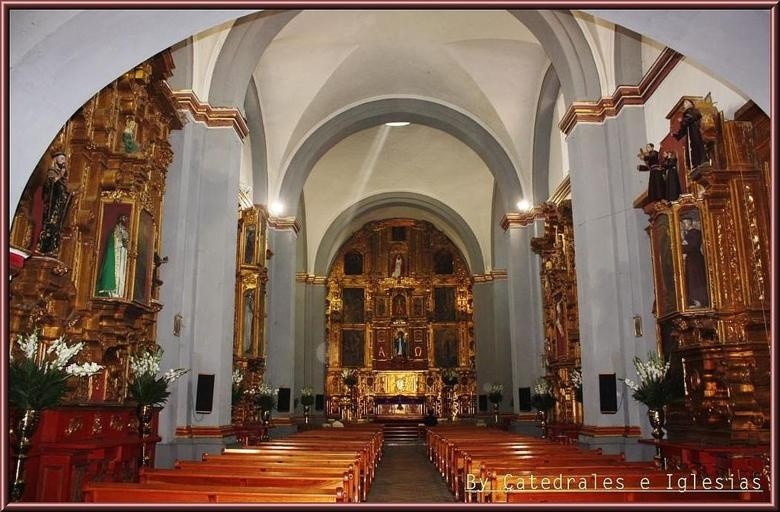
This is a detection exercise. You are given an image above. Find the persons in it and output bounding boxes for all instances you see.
[638,143,682,202]
[393,330,407,356]
[670,99,706,171]
[34,146,72,257]
[678,215,709,310]
[124,115,139,153]
[98,215,130,298]
[423,407,438,428]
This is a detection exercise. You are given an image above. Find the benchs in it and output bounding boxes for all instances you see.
[81,419,384,502]
[424,423,704,502]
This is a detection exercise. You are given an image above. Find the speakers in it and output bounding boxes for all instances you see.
[315,394,324,411]
[599,373,617,414]
[479,394,487,411]
[195,375,215,414]
[519,387,531,412]
[278,388,290,412]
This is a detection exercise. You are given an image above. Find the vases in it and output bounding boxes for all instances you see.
[261,407,272,424]
[537,409,550,428]
[136,401,153,435]
[304,404,309,416]
[648,406,665,439]
[493,403,499,413]
[14,407,40,453]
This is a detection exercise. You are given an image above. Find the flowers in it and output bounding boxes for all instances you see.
[10,325,107,410]
[301,386,314,405]
[531,380,555,409]
[488,384,504,402]
[125,349,190,405]
[621,353,681,410]
[256,383,279,409]
